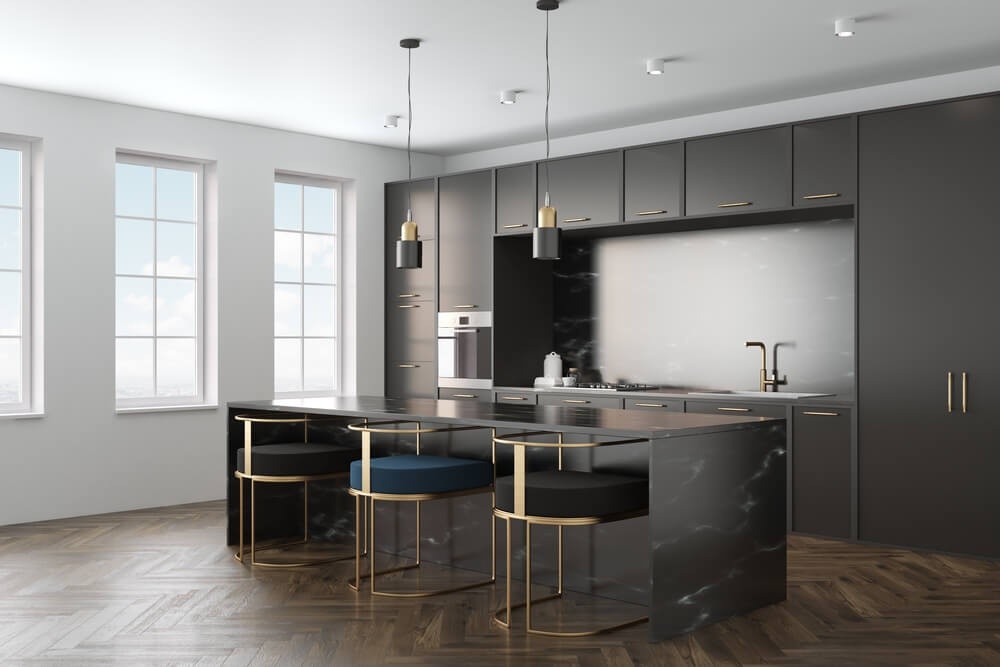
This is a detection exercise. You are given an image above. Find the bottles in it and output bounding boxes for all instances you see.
[543,352,562,378]
[569,367,579,385]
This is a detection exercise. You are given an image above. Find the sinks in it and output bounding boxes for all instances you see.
[687,389,838,400]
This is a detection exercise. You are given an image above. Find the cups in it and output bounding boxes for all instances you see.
[562,376,576,387]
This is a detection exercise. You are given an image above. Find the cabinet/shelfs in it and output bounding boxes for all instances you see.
[493,390,537,406]
[384,241,438,300]
[437,167,495,312]
[385,300,437,362]
[384,176,437,242]
[537,392,621,410]
[623,138,683,224]
[621,396,684,412]
[683,123,791,219]
[494,165,535,236]
[437,387,493,402]
[384,361,438,398]
[682,399,786,418]
[535,147,623,231]
[854,91,1000,558]
[791,112,854,208]
[788,403,855,544]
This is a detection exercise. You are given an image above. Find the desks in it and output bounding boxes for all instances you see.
[227,395,788,645]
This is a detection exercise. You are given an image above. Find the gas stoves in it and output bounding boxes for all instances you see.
[575,381,659,391]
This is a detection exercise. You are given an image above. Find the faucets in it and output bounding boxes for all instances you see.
[744,342,777,392]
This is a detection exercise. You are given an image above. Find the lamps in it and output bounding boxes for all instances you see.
[394,38,422,272]
[646,59,664,75]
[498,90,517,104]
[533,0,560,263]
[834,19,857,38]
[384,115,399,129]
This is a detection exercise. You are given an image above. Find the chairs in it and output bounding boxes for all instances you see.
[487,432,648,639]
[231,414,371,569]
[346,420,498,599]
